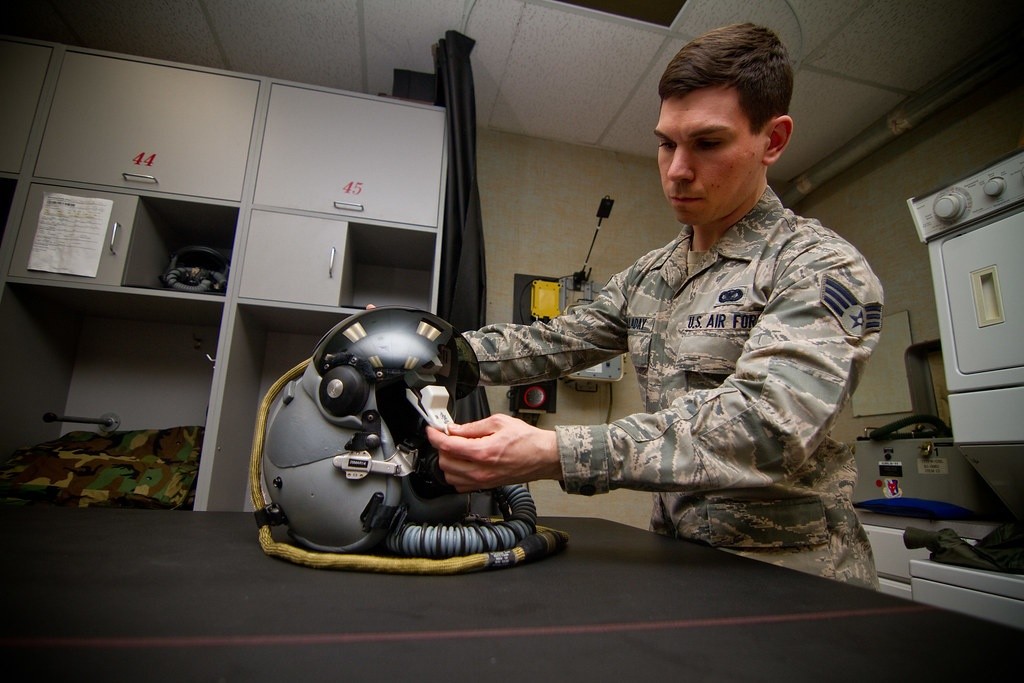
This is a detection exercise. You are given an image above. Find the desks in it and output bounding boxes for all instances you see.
[0,508,1024,683]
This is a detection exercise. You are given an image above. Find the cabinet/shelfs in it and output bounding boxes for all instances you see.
[0,34,448,512]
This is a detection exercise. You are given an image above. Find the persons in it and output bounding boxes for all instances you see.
[424,22,885,592]
